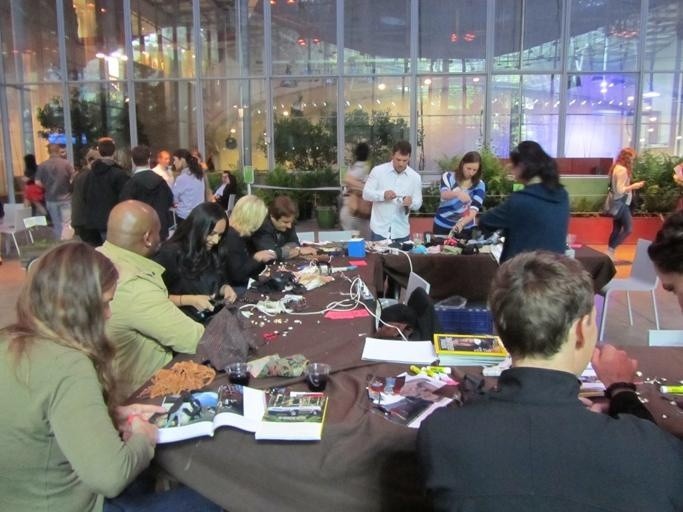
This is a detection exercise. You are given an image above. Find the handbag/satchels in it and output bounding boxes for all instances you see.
[349,193,371,219]
[602,190,629,219]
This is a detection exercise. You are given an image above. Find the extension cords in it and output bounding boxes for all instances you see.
[354,281,375,302]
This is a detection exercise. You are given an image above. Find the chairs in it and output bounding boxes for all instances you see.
[318,231,352,242]
[596,238,659,342]
[226,194,236,215]
[0,207,34,256]
[297,232,314,243]
[4,204,25,255]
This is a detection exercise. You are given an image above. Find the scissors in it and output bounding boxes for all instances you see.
[127,413,159,425]
[205,293,231,313]
[263,329,288,341]
[466,199,473,217]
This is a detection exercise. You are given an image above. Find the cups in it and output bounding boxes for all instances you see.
[224,363,252,385]
[413,233,422,245]
[308,362,329,393]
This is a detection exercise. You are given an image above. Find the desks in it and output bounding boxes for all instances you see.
[121,242,683,509]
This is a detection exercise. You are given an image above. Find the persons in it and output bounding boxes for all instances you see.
[0,243,167,511]
[674,160,682,214]
[410,250,683,509]
[88,200,207,405]
[648,211,683,316]
[606,147,646,257]
[26,136,318,319]
[432,151,485,241]
[477,143,570,265]
[341,143,371,240]
[363,141,423,247]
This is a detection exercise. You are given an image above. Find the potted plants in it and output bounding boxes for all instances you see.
[254,107,424,229]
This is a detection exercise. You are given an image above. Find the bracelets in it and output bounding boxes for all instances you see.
[605,381,636,400]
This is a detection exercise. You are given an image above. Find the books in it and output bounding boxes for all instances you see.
[254,389,329,442]
[157,384,267,446]
[433,333,510,367]
